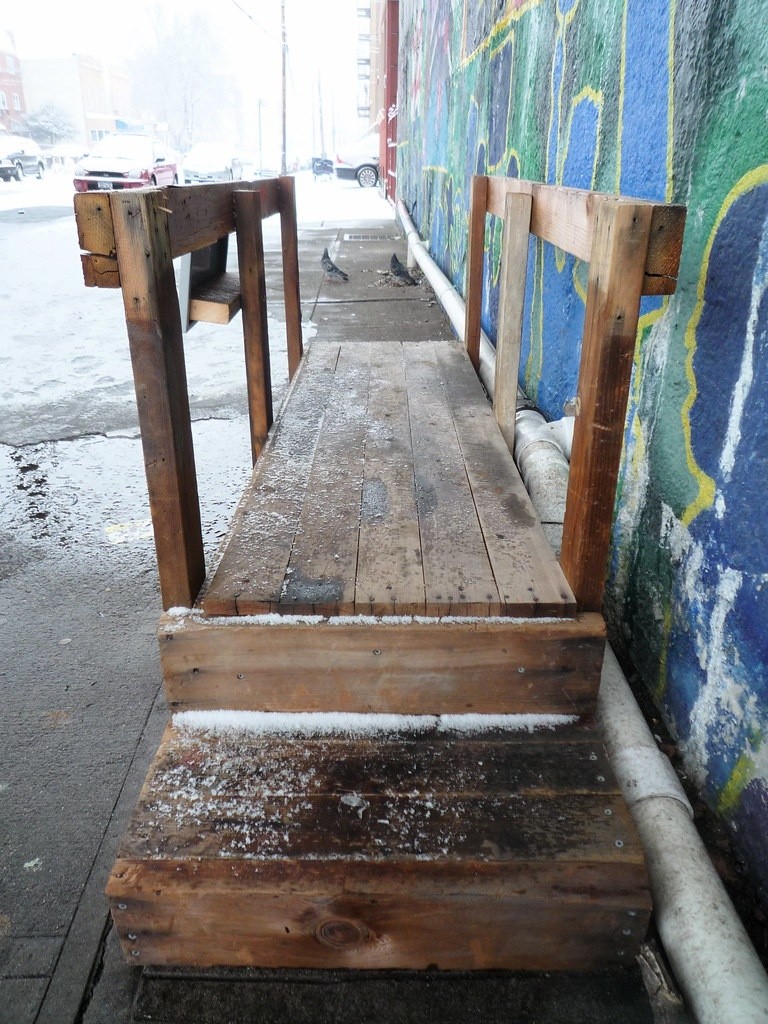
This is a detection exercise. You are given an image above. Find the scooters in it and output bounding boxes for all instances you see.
[312,155,333,176]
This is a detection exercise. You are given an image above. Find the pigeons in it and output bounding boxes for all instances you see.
[320,247,349,284]
[389,253,417,286]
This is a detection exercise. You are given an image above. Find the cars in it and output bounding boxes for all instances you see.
[177,144,242,186]
[66,130,180,193]
[0,132,49,184]
[333,134,381,189]
